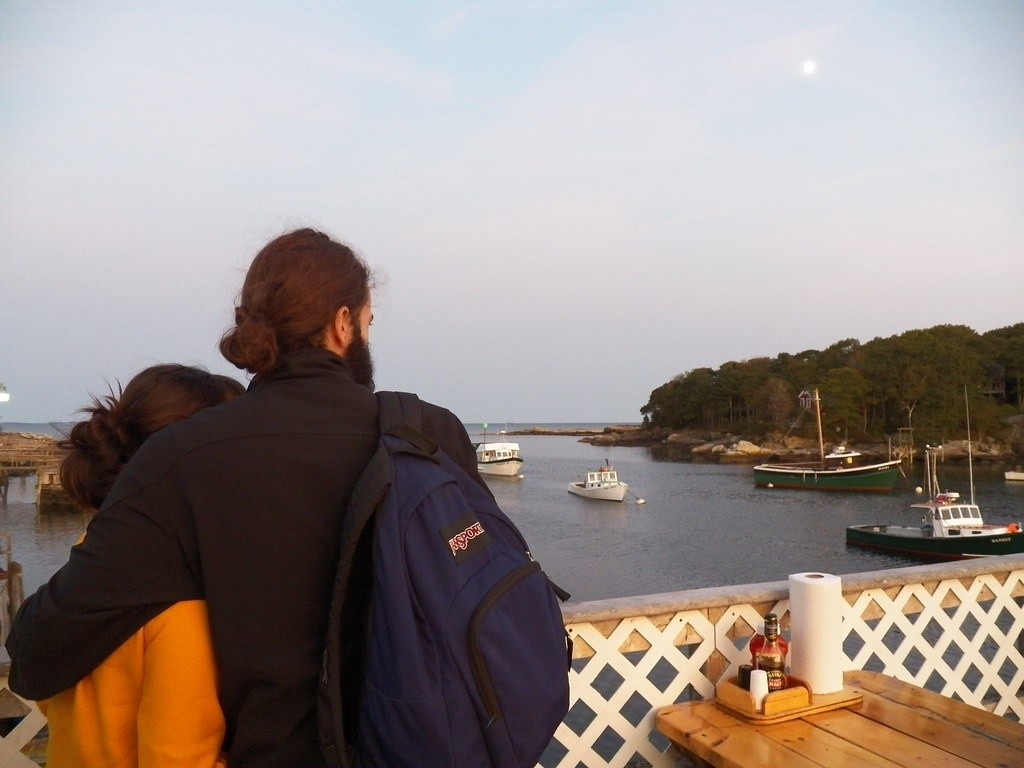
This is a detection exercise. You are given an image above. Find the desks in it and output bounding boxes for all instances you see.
[653,669,1023,768]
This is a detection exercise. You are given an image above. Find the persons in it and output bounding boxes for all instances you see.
[10,359,239,767]
[5,226,503,768]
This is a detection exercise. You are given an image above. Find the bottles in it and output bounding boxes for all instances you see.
[758,613,788,694]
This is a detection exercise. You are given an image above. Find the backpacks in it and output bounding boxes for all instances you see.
[313,390,574,768]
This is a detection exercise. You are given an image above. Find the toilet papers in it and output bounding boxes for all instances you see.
[787,568,847,696]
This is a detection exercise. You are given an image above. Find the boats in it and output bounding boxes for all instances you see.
[752,388,904,492]
[568,444,628,502]
[473,423,524,477]
[36,466,82,513]
[846,382,1023,562]
[1004,465,1024,480]
